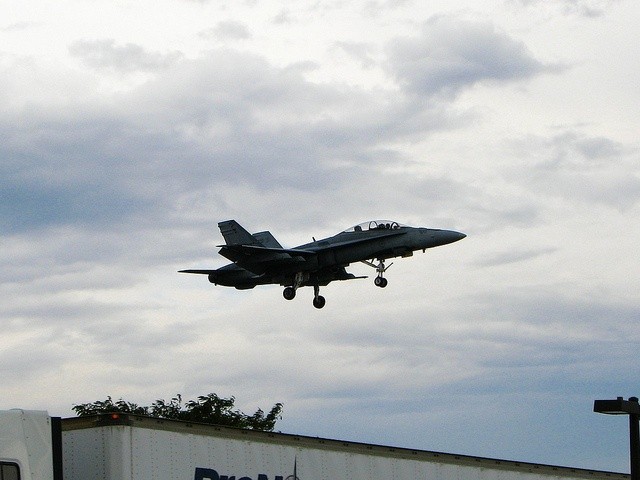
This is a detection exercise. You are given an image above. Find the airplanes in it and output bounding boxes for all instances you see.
[177,220,467,309]
